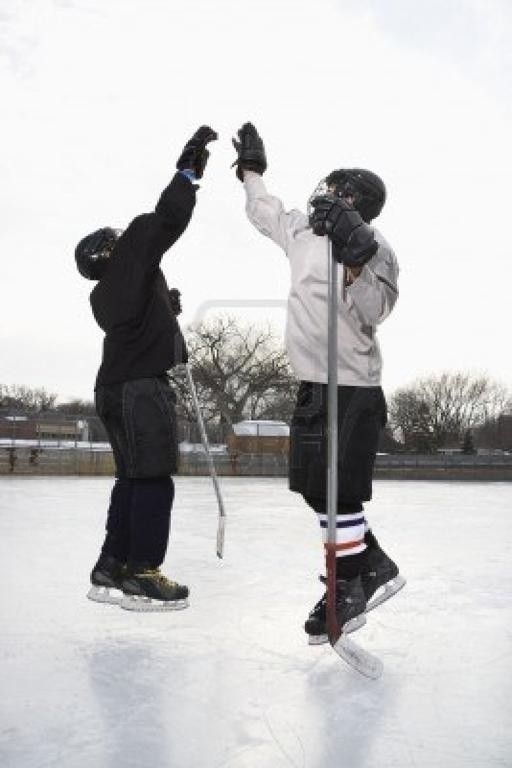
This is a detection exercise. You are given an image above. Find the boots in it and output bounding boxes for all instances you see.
[304,575,367,634]
[122,565,190,602]
[357,529,400,599]
[89,549,125,591]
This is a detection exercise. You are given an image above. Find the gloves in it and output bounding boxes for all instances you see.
[169,287,182,316]
[311,195,378,265]
[176,124,217,178]
[232,121,267,181]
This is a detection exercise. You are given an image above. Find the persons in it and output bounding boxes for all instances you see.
[76,122,218,613]
[233,122,404,647]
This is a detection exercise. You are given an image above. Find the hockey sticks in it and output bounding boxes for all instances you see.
[326,237,386,680]
[184,362,225,559]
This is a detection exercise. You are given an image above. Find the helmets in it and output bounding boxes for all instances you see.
[73,226,123,281]
[309,168,386,236]
